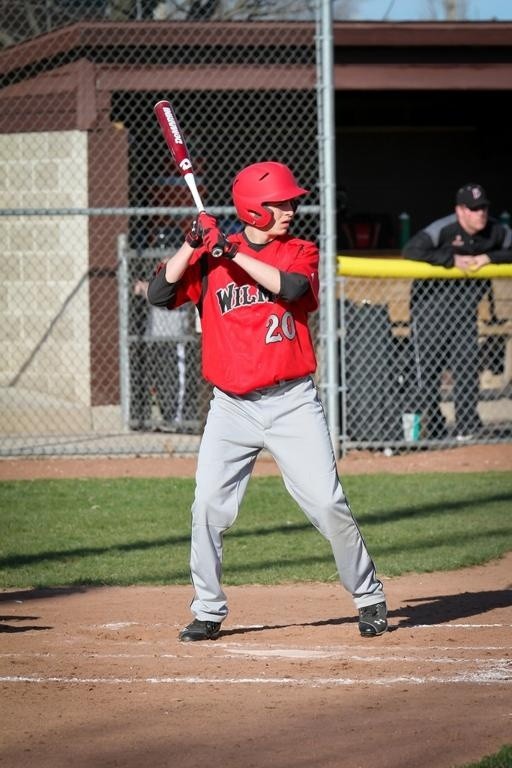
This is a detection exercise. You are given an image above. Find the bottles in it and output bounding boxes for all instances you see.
[454,184,490,208]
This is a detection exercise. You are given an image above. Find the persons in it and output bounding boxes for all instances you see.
[403,185,512,439]
[148,162,388,641]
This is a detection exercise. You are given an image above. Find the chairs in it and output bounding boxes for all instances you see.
[179,618,222,642]
[355,600,388,637]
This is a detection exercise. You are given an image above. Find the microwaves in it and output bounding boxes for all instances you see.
[153,99,224,259]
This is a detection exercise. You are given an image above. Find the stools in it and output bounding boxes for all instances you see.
[215,379,301,401]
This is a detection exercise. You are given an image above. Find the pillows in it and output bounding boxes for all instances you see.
[229,159,309,230]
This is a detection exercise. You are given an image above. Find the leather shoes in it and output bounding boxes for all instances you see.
[202,228,238,258]
[184,211,215,247]
